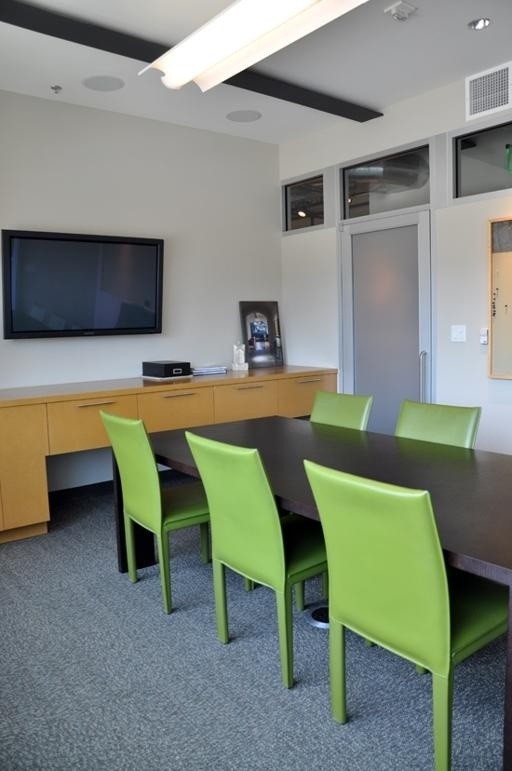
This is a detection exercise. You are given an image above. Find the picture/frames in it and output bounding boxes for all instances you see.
[238,299,285,369]
[484,214,511,382]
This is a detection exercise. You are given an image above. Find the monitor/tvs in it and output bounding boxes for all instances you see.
[0,228,165,341]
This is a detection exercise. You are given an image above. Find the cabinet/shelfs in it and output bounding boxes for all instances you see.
[46,379,139,454]
[212,369,280,424]
[276,366,340,419]
[1,389,50,545]
[136,377,216,435]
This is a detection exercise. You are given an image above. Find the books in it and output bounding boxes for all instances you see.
[192,367,227,375]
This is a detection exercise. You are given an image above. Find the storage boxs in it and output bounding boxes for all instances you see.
[141,360,191,377]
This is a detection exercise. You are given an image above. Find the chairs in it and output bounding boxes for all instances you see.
[394,400,483,455]
[98,407,256,618]
[306,388,374,431]
[184,431,378,690]
[302,456,509,771]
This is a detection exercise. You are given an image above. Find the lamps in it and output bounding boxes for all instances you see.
[135,1,370,95]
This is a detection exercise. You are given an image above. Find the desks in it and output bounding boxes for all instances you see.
[110,415,510,771]
